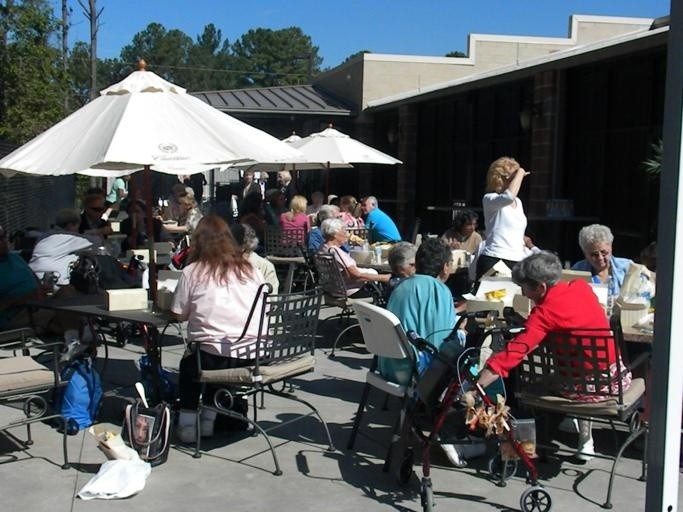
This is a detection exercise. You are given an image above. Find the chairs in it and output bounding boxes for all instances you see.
[189,282,336,476]
[445,268,471,302]
[344,220,375,240]
[0,325,73,470]
[346,300,421,476]
[500,325,653,509]
[32,270,60,297]
[296,243,318,286]
[310,250,374,358]
[403,216,422,245]
[262,220,316,296]
[360,280,388,309]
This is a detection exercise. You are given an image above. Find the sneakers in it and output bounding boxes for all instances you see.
[200,420,214,437]
[175,423,197,443]
[576,434,596,460]
[440,444,468,467]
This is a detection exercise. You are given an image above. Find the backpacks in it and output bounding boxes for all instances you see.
[53,354,105,433]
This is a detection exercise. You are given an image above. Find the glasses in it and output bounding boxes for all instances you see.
[88,207,107,213]
[410,263,416,266]
[592,250,609,256]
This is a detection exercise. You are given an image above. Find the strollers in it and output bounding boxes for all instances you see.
[401,311,551,512]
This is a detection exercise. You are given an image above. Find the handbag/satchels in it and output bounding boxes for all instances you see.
[64,241,147,297]
[119,402,171,466]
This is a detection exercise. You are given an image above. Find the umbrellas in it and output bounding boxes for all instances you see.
[0,59,307,301]
[230,131,354,198]
[288,121,404,206]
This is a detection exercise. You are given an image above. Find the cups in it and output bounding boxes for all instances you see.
[145,301,153,312]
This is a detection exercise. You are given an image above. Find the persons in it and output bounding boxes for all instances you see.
[274,170,296,206]
[317,218,393,296]
[27,210,104,292]
[172,216,270,446]
[569,224,656,336]
[472,157,530,295]
[229,221,280,305]
[105,174,132,207]
[82,187,119,210]
[0,226,102,363]
[79,193,114,240]
[467,251,632,460]
[164,193,202,234]
[232,170,263,222]
[385,241,420,296]
[439,209,483,255]
[120,205,169,245]
[376,237,494,384]
[184,172,208,202]
[359,197,402,246]
[280,195,311,246]
[338,197,363,228]
[307,205,350,262]
